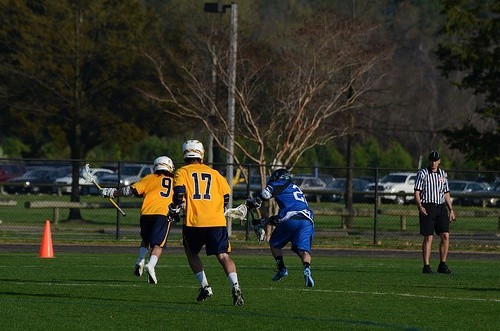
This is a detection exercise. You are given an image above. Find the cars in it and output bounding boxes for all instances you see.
[98,164,154,198]
[297,174,335,184]
[447,181,500,208]
[324,178,371,205]
[0,164,26,183]
[232,173,271,199]
[2,167,56,195]
[54,169,115,197]
[293,177,328,202]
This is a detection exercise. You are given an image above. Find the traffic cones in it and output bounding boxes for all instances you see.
[38,219,56,258]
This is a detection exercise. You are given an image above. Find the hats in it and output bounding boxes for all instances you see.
[428,151,440,161]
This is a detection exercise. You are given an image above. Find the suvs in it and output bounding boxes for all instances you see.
[363,172,420,205]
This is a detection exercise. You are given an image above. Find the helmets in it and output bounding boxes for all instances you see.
[182,139,205,164]
[153,156,174,173]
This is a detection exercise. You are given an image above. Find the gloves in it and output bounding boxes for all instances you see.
[99,188,116,199]
[246,196,263,211]
[268,214,280,226]
[166,203,180,223]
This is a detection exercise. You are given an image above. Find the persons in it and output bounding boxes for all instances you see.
[414,151,456,275]
[99,155,191,284]
[230,169,315,287]
[171,139,245,307]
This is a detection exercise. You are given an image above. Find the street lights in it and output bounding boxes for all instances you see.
[202,0,238,237]
[341,81,360,229]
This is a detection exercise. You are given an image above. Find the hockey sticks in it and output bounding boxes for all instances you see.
[249,209,266,245]
[83,164,126,217]
[180,203,247,221]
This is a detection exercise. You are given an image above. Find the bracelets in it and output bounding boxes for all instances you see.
[449,208,453,210]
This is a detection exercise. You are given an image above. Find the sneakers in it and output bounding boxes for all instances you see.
[271,268,288,282]
[436,264,451,274]
[197,286,213,302]
[303,267,314,287]
[144,263,157,285]
[423,266,433,274]
[231,286,245,308]
[134,257,145,276]
